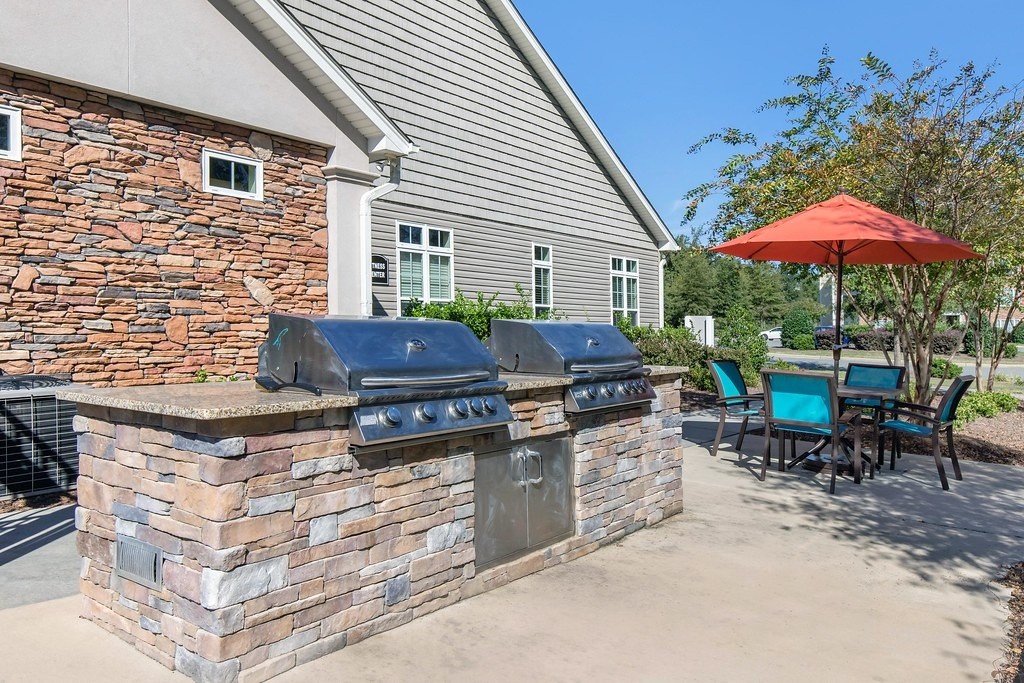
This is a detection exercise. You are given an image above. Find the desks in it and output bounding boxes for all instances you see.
[836,383,904,480]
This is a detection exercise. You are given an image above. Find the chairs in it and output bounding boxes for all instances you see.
[839,362,906,465]
[707,358,796,472]
[880,374,975,490]
[759,367,863,493]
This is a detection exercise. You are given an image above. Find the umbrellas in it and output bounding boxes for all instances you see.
[709,194,986,474]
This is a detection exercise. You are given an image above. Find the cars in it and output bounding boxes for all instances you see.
[759,327,783,340]
[812,326,857,348]
